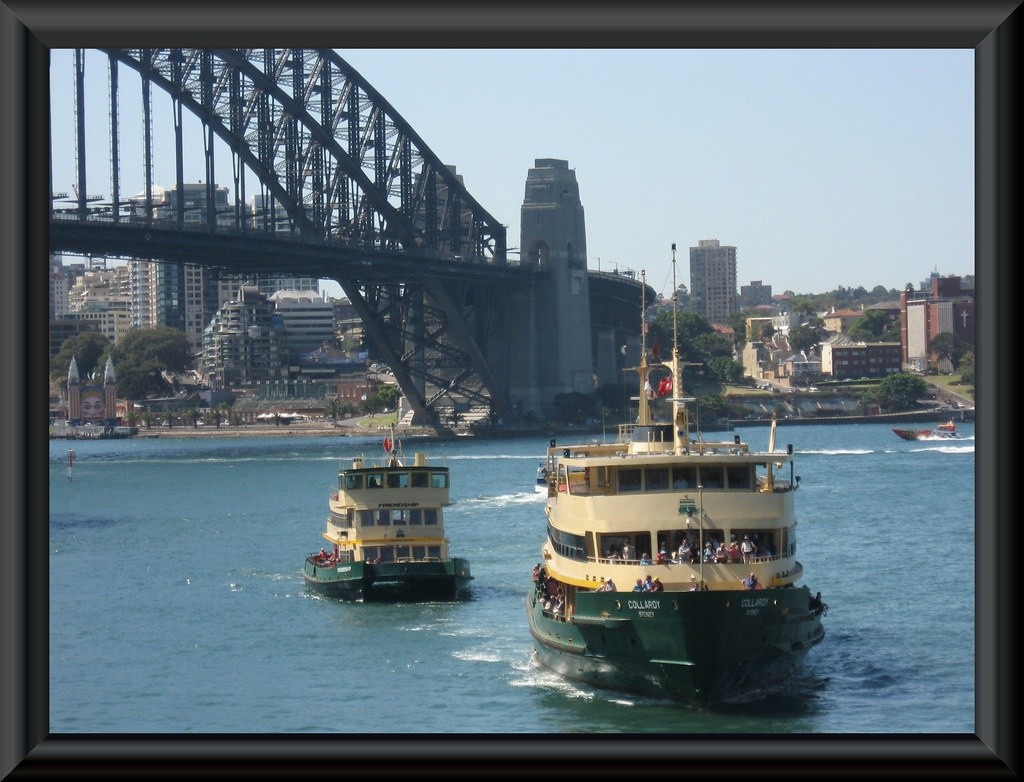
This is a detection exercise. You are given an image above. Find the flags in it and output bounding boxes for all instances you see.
[384,437,392,452]
[657,375,672,398]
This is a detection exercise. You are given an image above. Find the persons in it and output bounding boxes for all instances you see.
[632,575,663,593]
[607,545,618,564]
[640,553,651,566]
[319,547,336,562]
[657,534,772,564]
[347,476,356,488]
[532,563,565,618]
[674,474,689,488]
[742,573,757,590]
[366,557,381,564]
[606,579,617,592]
[690,580,708,591]
[622,539,636,565]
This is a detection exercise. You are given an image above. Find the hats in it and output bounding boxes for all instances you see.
[653,577,660,583]
[607,578,612,581]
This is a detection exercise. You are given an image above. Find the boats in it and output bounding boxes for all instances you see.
[530,243,827,707]
[302,423,474,600]
[892,418,959,441]
